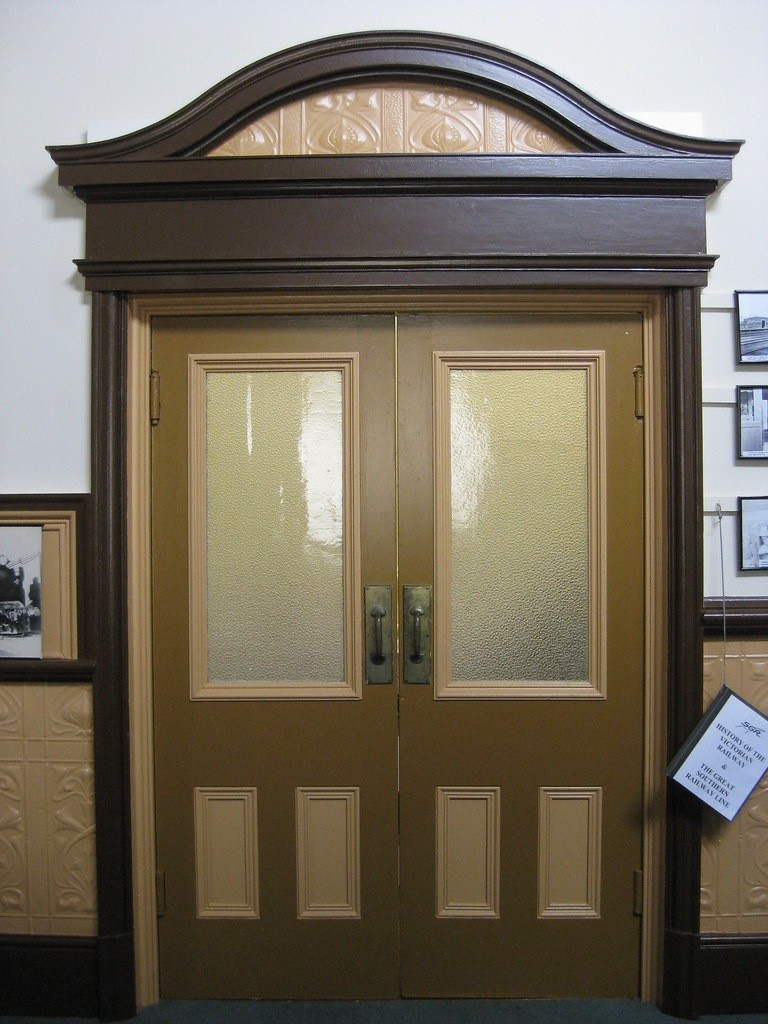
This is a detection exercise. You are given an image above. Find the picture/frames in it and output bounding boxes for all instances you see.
[0,494,98,682]
[734,290,768,362]
[739,496,768,570]
[737,385,768,460]
